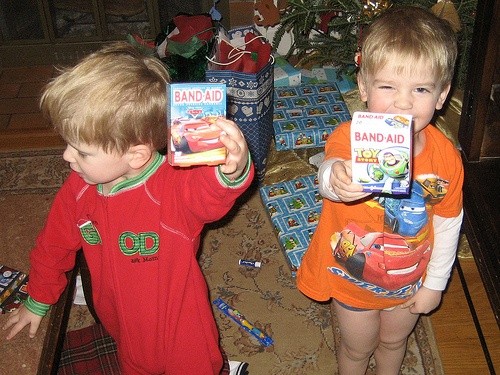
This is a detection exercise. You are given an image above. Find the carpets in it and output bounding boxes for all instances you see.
[0,147,442,375]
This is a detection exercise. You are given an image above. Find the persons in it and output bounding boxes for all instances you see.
[2,42,255,375]
[295,5,465,375]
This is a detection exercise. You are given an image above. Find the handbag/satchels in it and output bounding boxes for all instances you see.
[154,13,219,83]
[205,25,274,183]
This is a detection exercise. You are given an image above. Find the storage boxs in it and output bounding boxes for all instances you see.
[271,83,353,152]
[165,81,227,168]
[273,53,302,87]
[348,111,412,196]
[259,174,324,277]
